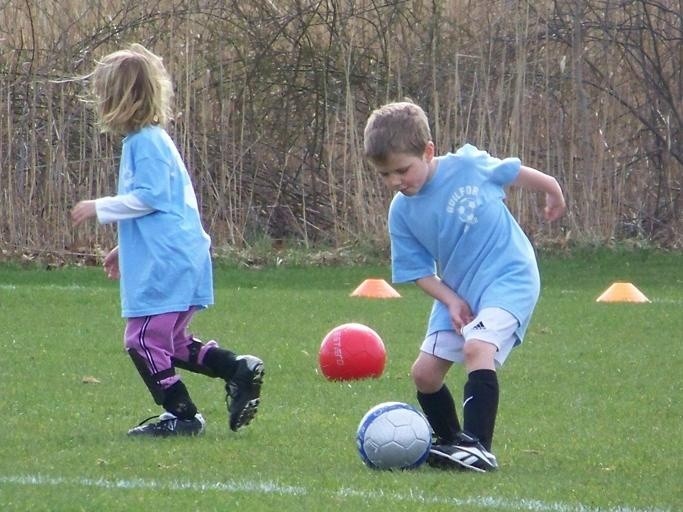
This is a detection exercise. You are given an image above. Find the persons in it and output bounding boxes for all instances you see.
[363,101,566,475]
[70,41,265,439]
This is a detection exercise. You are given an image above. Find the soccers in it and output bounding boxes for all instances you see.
[319,323,385,380]
[357,401,431,470]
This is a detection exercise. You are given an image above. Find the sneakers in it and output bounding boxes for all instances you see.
[128,411,207,437]
[225,355,264,432]
[426,430,497,473]
